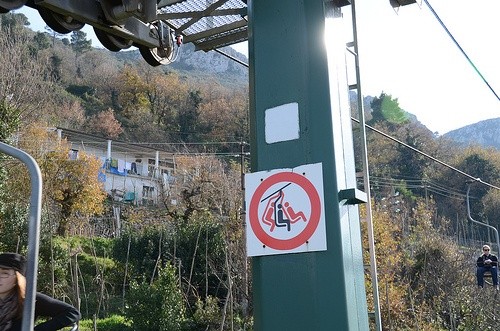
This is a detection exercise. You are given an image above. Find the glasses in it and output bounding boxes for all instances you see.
[0,273,16,278]
[484,249,490,251]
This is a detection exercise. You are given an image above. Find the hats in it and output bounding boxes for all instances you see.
[0,252,26,276]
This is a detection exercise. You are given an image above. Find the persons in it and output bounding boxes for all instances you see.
[476,245,499,296]
[0,252,81,331]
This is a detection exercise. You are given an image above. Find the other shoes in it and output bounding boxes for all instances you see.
[494,285,499,291]
[478,286,482,290]
